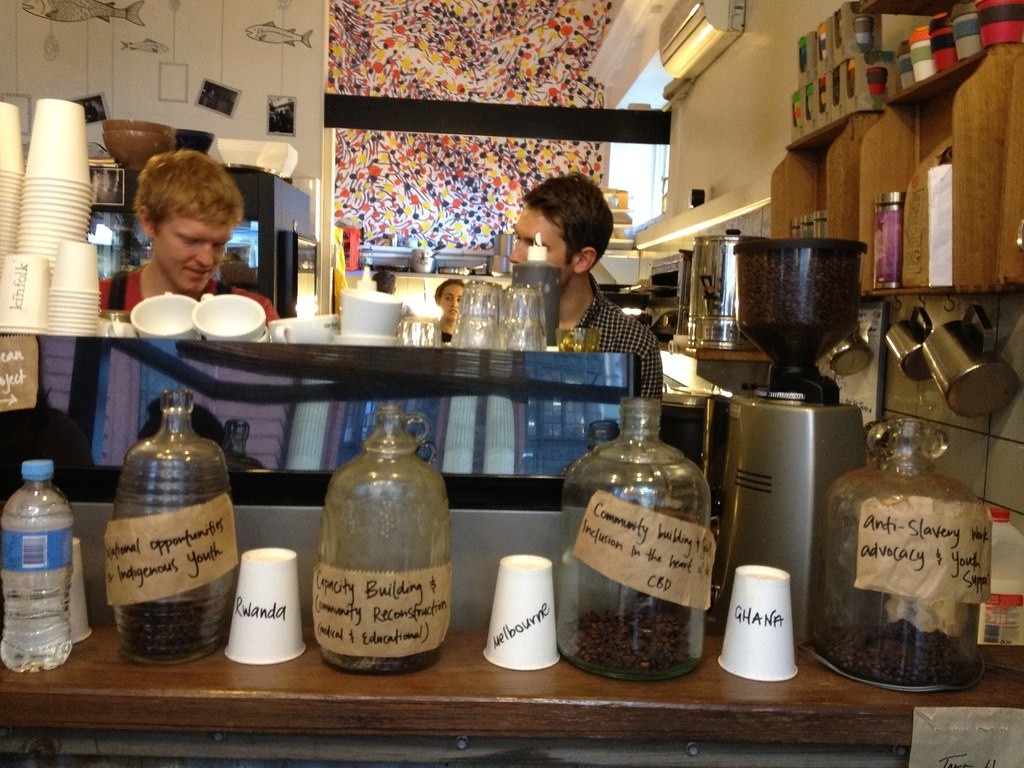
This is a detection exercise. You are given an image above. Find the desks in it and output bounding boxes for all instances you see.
[0,619,1024,768]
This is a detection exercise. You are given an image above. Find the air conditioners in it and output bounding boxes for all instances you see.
[659,0,747,81]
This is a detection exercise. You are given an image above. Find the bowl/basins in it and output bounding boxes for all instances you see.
[101,119,176,171]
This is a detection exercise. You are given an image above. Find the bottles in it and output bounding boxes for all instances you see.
[873,191,906,289]
[805,417,989,693]
[988,508,1024,606]
[106,391,238,665]
[556,397,712,679]
[312,404,453,672]
[0,459,75,675]
[790,209,827,238]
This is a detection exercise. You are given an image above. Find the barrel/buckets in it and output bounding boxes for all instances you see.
[674,230,757,351]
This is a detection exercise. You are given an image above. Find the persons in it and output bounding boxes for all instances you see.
[508,174,663,399]
[435,278,464,342]
[98,148,279,323]
[373,271,414,320]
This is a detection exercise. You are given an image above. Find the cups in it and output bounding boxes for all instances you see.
[224,547,306,666]
[717,563,798,682]
[555,327,600,353]
[885,305,932,382]
[411,249,433,273]
[483,554,560,671]
[68,538,92,644]
[101,281,546,351]
[824,324,872,377]
[921,302,1020,416]
[485,233,513,274]
[0,97,101,337]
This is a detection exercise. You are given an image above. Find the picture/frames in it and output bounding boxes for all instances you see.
[158,62,191,102]
[195,78,242,119]
[266,94,300,138]
[69,92,112,123]
